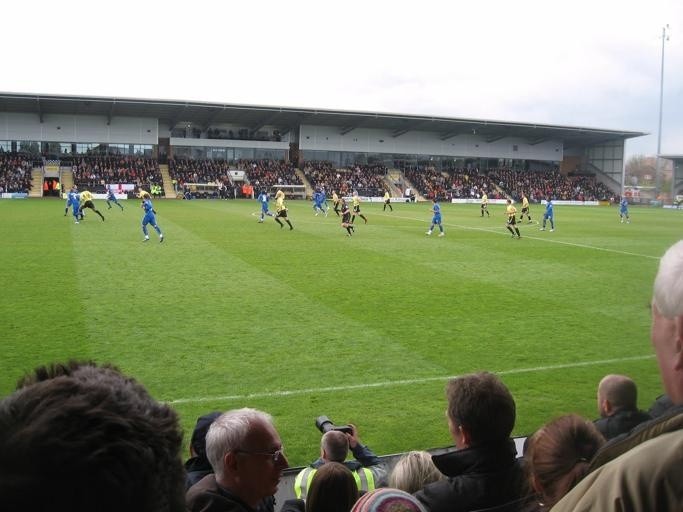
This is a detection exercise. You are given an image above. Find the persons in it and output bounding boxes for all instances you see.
[257,187,293,231]
[168,158,306,201]
[479,191,490,217]
[540,196,554,232]
[64,185,104,224]
[518,194,532,224]
[106,184,124,211]
[400,165,621,204]
[0,357,683,512]
[549,240,683,507]
[137,188,164,243]
[301,159,391,200]
[619,197,630,224]
[0,147,165,198]
[383,190,393,211]
[311,187,367,237]
[424,198,444,237]
[676,193,682,209]
[505,200,520,239]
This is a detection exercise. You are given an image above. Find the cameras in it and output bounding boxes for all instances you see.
[315,415,353,436]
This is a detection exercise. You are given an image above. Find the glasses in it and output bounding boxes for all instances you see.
[240,447,284,460]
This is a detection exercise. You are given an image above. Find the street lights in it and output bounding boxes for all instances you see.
[652,22,674,204]
